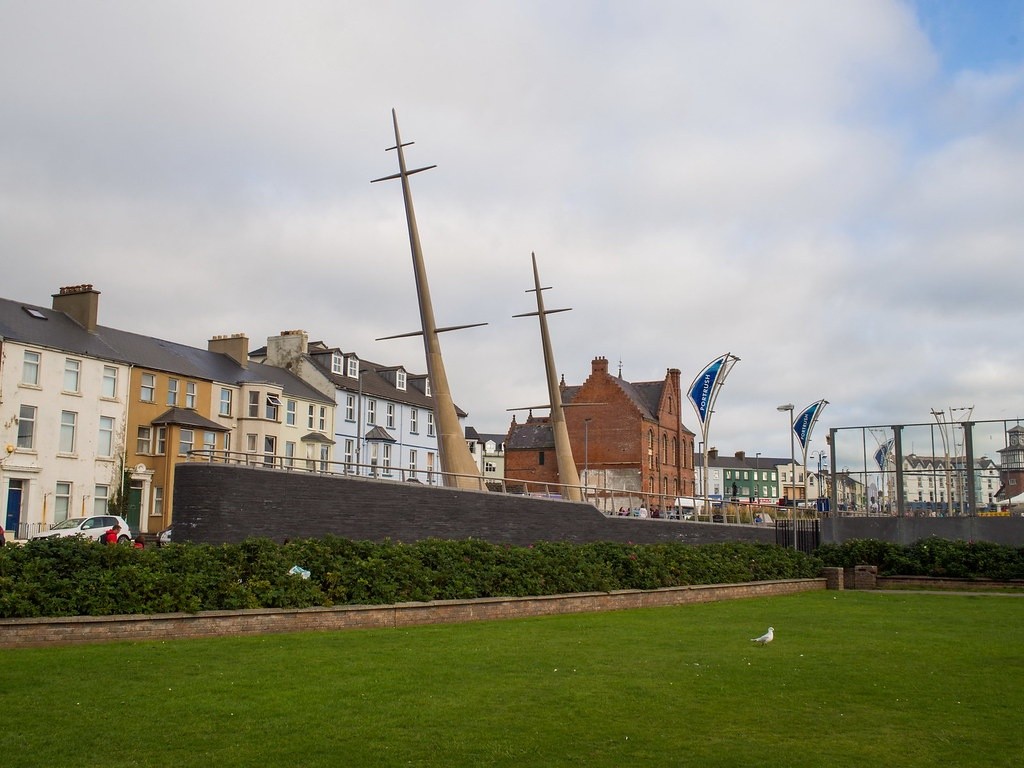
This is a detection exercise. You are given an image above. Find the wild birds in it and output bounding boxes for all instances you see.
[751,626,775,648]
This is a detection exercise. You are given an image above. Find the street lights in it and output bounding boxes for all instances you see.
[582,417,594,503]
[777,401,798,551]
[755,452,762,504]
[698,440,704,497]
[809,450,827,498]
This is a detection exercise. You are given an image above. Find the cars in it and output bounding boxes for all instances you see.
[30,514,132,547]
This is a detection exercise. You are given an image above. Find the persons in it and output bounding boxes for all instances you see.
[755,514,762,522]
[633,507,638,516]
[651,508,660,518]
[638,504,647,518]
[626,508,635,516]
[133,535,146,550]
[105,525,122,545]
[619,507,625,516]
[838,499,843,511]
